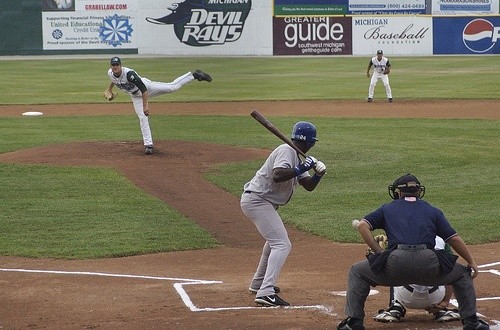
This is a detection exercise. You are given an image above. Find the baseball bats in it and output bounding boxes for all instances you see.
[250,110,326,175]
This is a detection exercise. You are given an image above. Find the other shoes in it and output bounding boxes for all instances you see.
[194,70,211,81]
[368,98,372,102]
[145,147,152,153]
[389,98,392,102]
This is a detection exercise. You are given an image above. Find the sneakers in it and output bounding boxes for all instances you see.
[436,310,461,322]
[372,310,401,323]
[337,317,364,330]
[255,294,289,306]
[463,317,489,330]
[249,285,280,295]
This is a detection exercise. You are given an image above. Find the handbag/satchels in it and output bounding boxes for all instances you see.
[365,245,398,274]
[431,247,459,274]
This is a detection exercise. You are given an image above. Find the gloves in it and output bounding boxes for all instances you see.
[303,155,318,170]
[312,160,326,176]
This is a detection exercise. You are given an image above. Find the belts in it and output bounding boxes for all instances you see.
[132,89,138,94]
[403,285,439,294]
[396,244,427,250]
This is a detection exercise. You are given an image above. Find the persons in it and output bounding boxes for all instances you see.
[104,57,213,155]
[367,50,393,103]
[240,121,328,307]
[336,174,490,330]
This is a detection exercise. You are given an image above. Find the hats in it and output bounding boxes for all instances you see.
[377,50,383,54]
[291,121,319,147]
[111,57,120,65]
[397,174,419,193]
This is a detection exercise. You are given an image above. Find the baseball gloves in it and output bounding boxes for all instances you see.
[384,66,390,74]
[365,235,389,256]
[103,90,118,100]
